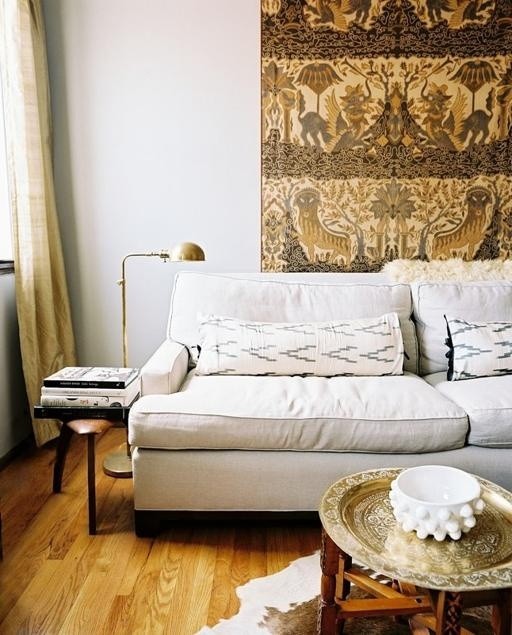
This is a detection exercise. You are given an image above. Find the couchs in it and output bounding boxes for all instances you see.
[129,271,512,538]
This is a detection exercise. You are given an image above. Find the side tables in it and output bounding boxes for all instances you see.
[51,415,128,536]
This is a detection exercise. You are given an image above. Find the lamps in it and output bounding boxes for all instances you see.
[103,239,206,480]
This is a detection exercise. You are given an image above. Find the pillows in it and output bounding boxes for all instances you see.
[185,316,407,380]
[440,312,512,379]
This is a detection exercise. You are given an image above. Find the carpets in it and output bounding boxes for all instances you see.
[190,547,413,633]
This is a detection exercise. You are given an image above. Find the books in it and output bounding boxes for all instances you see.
[40,390,140,407]
[40,375,141,396]
[44,366,139,389]
